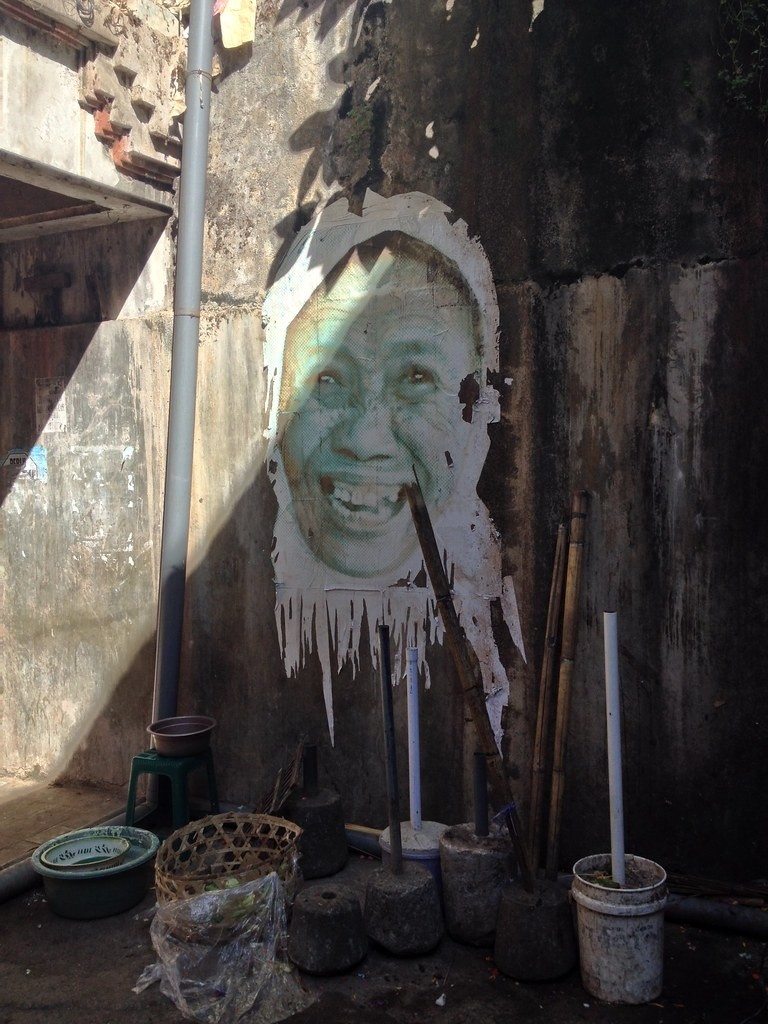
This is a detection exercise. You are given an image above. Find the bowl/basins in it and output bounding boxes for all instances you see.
[30,825,160,920]
[40,835,131,873]
[146,715,217,758]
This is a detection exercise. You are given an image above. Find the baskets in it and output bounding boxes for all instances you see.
[156,811,303,942]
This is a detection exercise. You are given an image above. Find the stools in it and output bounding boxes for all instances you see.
[125,748,220,863]
